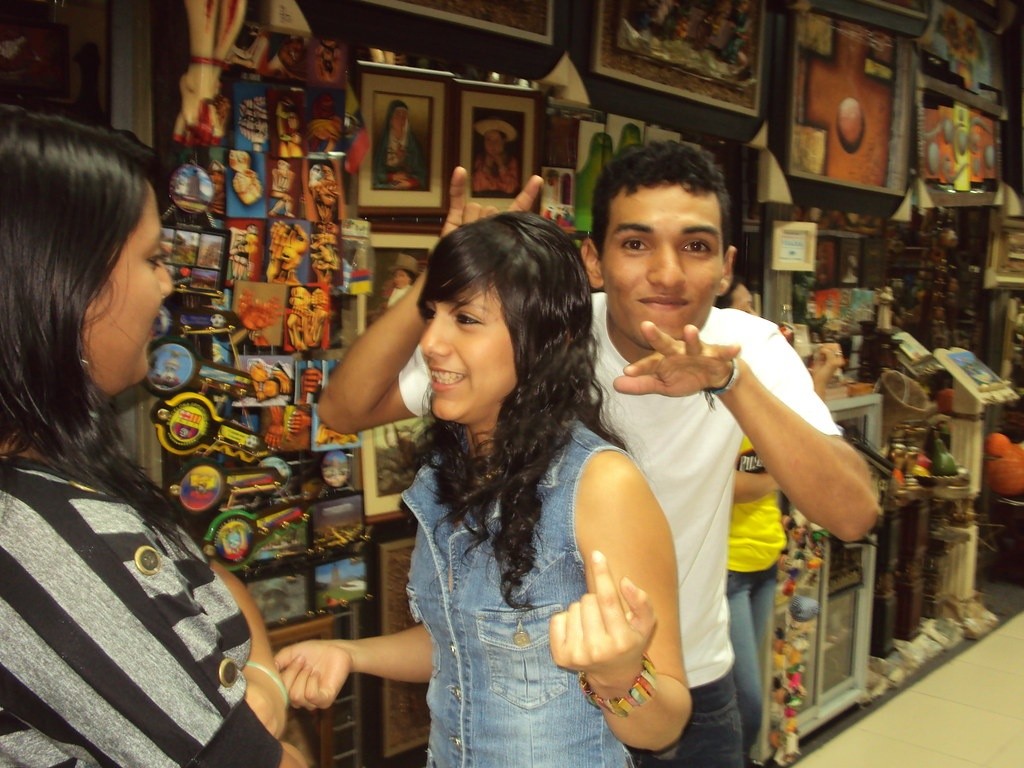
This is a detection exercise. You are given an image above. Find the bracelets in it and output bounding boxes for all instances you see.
[246,660,288,706]
[579,652,657,718]
[709,359,738,395]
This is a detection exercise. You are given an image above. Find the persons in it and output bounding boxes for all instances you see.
[0,105,314,768]
[275,210,692,768]
[317,139,879,768]
[714,277,846,750]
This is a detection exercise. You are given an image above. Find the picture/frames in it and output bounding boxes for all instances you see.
[449,77,541,213]
[329,223,446,349]
[354,70,451,218]
[361,0,553,45]
[377,539,432,758]
[591,0,765,118]
[265,612,336,768]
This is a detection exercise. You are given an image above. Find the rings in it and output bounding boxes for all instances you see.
[836,351,843,356]
